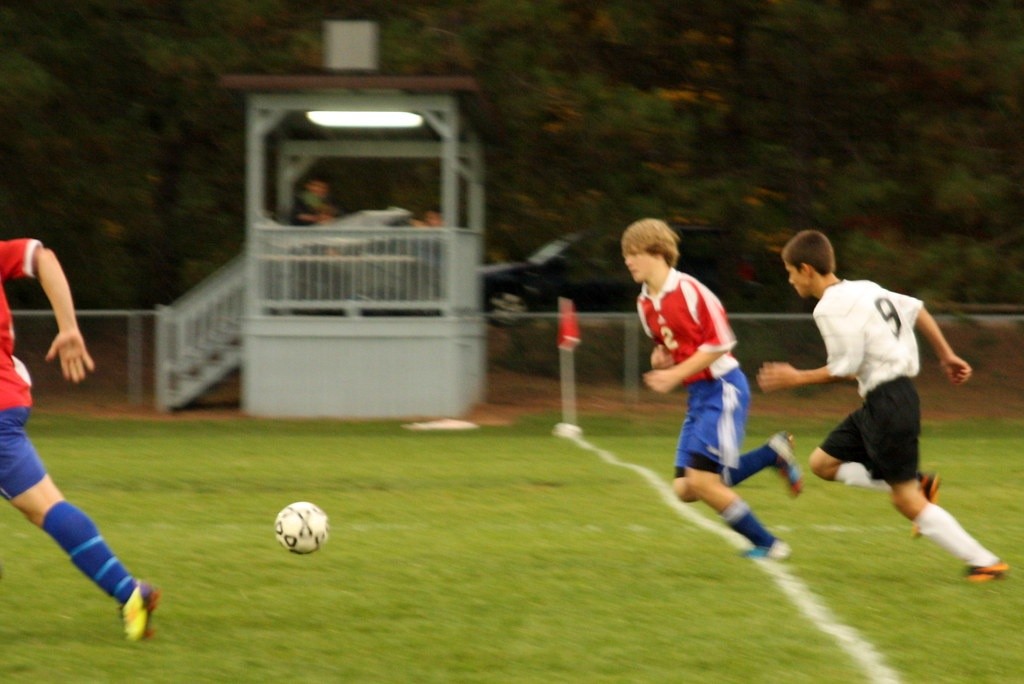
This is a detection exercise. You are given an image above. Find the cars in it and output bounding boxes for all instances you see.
[483,213,787,310]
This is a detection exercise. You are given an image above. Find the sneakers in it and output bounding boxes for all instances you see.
[962,559,1011,582]
[911,470,942,537]
[771,430,805,498]
[741,541,791,568]
[120,577,159,644]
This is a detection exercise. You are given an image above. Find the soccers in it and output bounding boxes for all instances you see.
[275,501,329,554]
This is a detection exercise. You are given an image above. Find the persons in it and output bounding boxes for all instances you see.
[0,237,164,642]
[756,230,1011,584]
[0,0,1024,313]
[621,218,805,563]
